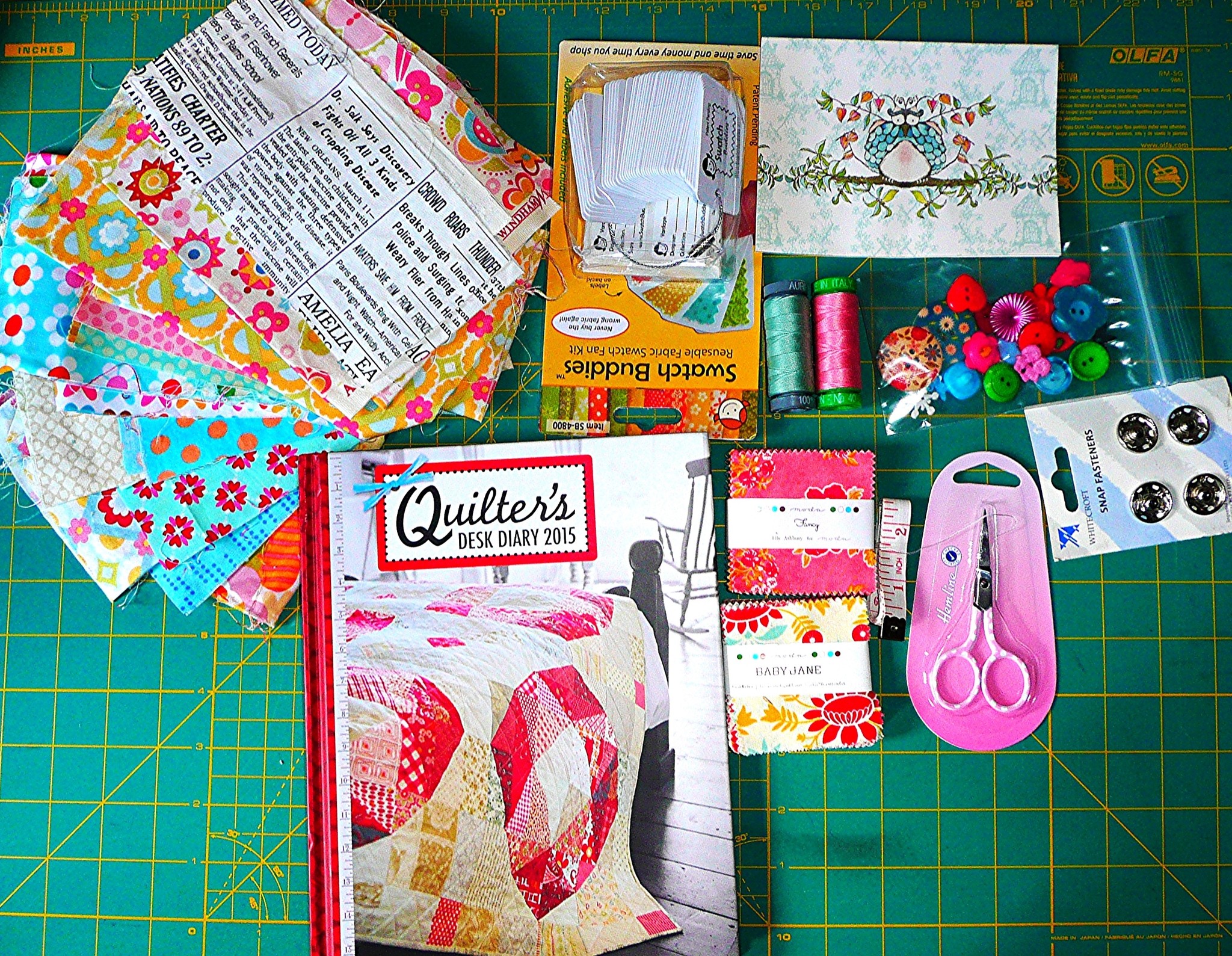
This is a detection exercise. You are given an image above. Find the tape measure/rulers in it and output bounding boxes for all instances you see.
[865,497,910,642]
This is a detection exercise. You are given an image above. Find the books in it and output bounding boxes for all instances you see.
[296,434,740,956]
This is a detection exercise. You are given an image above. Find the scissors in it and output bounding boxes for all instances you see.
[929,510,1032,712]
